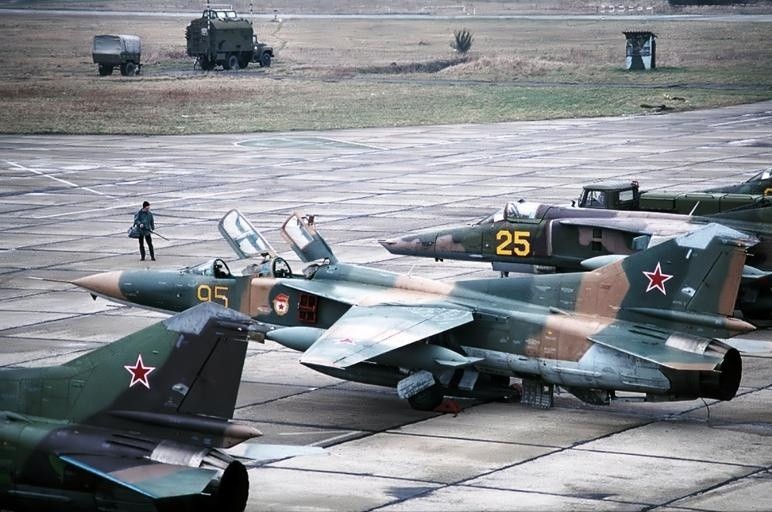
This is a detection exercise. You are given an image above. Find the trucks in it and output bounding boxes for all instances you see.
[92,9,275,75]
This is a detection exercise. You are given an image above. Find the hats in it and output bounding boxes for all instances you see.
[143,201,150,209]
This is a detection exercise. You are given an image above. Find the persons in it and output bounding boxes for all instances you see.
[134,201,156,261]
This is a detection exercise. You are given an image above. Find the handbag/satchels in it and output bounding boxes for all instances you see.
[127,227,141,238]
